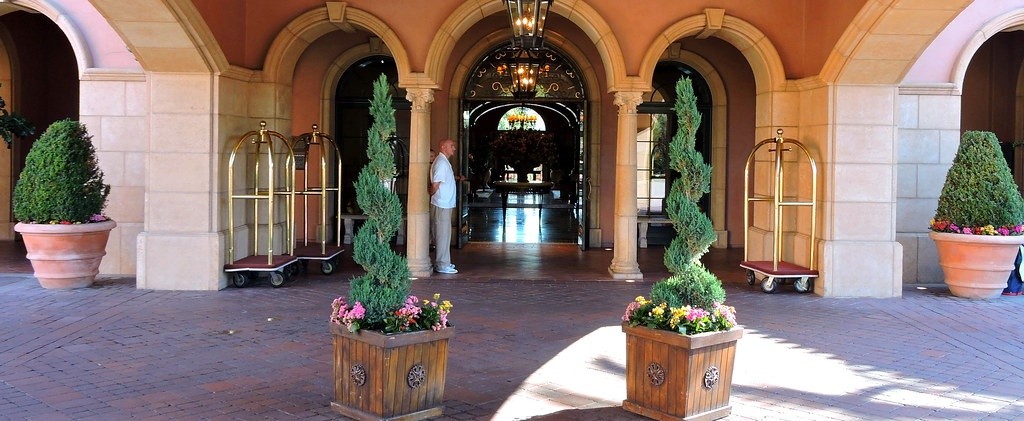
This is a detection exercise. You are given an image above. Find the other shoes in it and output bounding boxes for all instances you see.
[429,244,434,252]
[436,267,458,273]
[434,264,455,270]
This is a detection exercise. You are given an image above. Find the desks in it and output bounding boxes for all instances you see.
[493,181,553,220]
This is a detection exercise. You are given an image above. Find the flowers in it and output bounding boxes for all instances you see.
[330,73,453,334]
[9,118,110,225]
[622,74,736,332]
[487,128,557,167]
[927,130,1024,236]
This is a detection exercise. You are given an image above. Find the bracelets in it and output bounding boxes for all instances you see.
[457,174,462,180]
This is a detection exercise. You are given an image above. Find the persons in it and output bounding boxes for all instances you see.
[427,138,465,274]
[469,152,482,198]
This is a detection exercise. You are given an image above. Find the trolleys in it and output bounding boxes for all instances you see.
[221,120,299,288]
[739,128,821,294]
[284,124,347,276]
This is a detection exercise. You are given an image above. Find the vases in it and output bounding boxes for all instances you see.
[329,322,455,421]
[929,231,1024,299]
[509,164,533,181]
[12,221,117,290]
[620,323,743,421]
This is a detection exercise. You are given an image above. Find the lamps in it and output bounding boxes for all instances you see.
[506,109,536,127]
[501,43,543,108]
[502,0,553,44]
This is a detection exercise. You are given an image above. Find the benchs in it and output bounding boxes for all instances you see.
[637,216,674,248]
[335,214,407,247]
